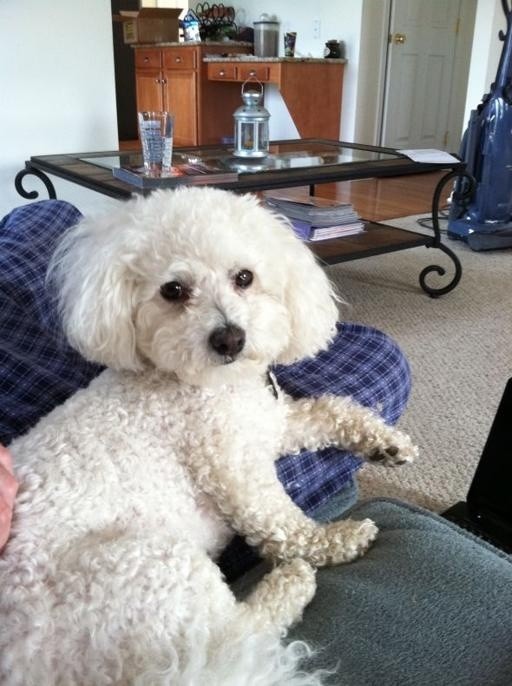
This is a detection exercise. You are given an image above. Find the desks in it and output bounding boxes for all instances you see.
[16,138,476,297]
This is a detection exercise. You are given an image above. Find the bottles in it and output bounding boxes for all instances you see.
[323,39,341,58]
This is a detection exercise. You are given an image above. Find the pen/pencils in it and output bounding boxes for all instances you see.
[222,53,254,57]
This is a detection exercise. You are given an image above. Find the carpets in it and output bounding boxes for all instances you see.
[320,212,512,513]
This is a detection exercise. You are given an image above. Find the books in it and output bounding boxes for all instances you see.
[266,194,368,244]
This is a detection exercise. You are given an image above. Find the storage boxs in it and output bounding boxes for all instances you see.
[112,7,183,45]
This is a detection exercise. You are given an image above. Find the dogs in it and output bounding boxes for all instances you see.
[0,186,417,686]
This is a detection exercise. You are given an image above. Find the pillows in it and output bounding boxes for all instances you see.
[225,494,511,686]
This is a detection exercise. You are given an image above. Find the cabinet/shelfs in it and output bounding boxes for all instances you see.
[203,58,346,142]
[133,44,264,147]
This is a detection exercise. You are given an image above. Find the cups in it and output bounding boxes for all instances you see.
[137,109,175,172]
[283,31,297,56]
[252,20,279,56]
